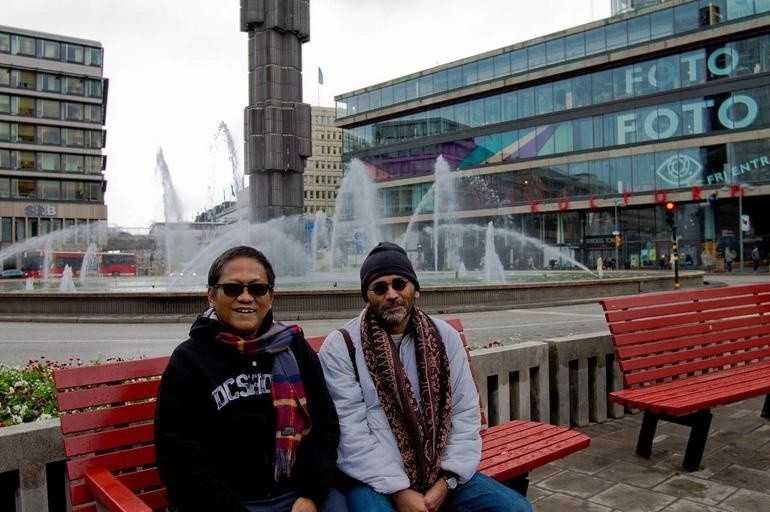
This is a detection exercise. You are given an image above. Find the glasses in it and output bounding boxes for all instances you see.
[214,283,272,297]
[368,278,410,295]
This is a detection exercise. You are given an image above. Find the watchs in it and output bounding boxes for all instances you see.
[439,469,458,491]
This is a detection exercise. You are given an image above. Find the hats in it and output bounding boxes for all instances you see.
[360,241,420,303]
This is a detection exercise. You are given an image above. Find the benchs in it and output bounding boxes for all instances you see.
[53,317,591,512]
[598,284,770,472]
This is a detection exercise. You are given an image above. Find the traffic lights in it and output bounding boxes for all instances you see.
[663,200,676,226]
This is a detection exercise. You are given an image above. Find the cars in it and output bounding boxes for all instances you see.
[0,270,25,279]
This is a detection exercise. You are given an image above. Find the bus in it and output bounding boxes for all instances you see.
[22,249,137,282]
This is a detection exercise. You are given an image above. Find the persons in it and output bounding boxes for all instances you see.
[660,252,665,269]
[725,247,737,271]
[690,244,698,266]
[152,244,349,512]
[700,248,713,272]
[317,243,536,512]
[685,251,693,265]
[669,250,675,275]
[752,246,764,271]
